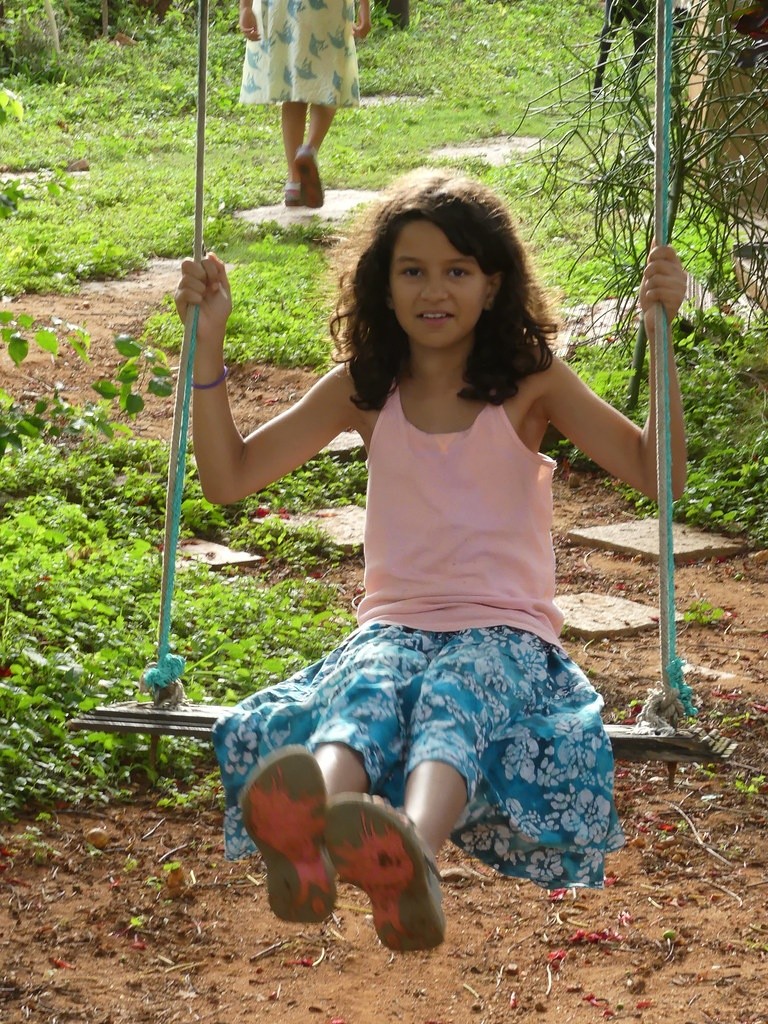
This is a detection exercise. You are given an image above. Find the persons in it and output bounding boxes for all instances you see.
[238,0,371,208]
[173,176,687,954]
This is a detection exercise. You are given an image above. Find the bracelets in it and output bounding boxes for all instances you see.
[191,366,228,389]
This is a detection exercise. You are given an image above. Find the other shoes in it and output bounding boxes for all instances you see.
[323,791,445,953]
[295,149,323,208]
[284,183,305,207]
[242,743,337,921]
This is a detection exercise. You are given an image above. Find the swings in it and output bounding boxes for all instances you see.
[68,0,739,777]
